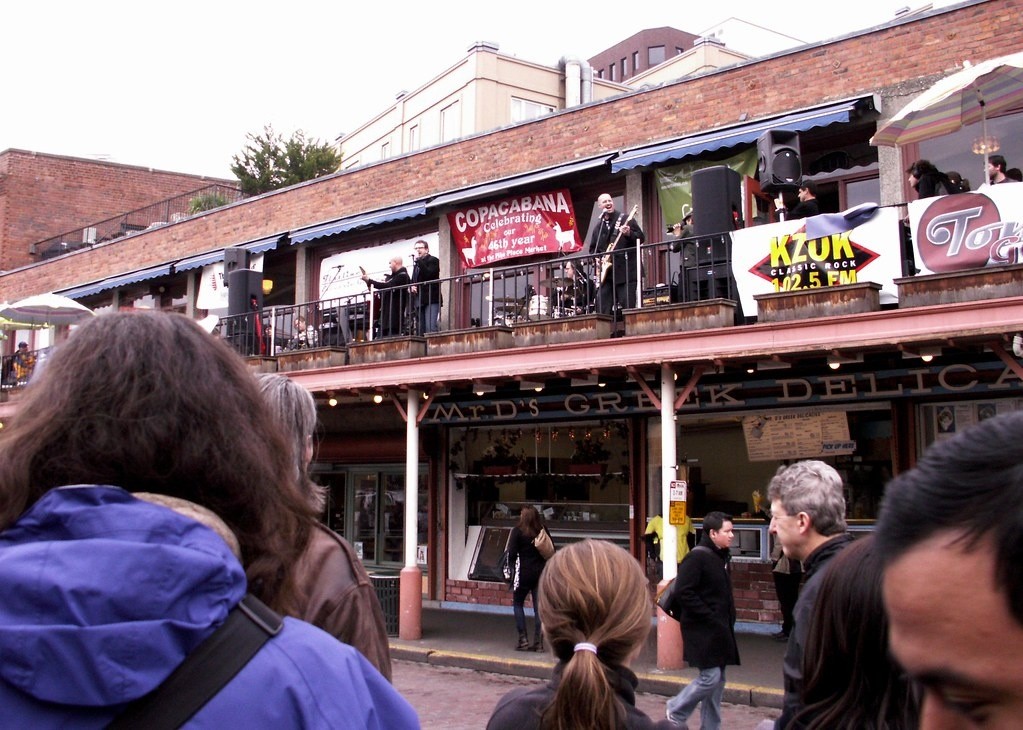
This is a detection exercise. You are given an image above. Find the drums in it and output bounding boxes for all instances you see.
[529,295,548,319]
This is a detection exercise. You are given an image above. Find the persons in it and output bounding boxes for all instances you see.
[411,240,444,332]
[259,315,319,354]
[904,154,962,200]
[644,512,697,569]
[0,312,422,728]
[875,411,1023,729]
[484,538,689,730]
[664,510,741,729]
[789,530,924,730]
[757,503,801,639]
[983,155,1020,184]
[244,374,394,687]
[564,178,826,316]
[360,255,410,335]
[768,459,854,730]
[504,506,557,652]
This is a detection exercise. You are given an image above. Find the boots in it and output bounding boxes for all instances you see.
[528,633,545,652]
[517,630,529,650]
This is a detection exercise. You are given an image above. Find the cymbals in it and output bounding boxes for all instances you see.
[497,297,525,303]
[539,276,575,286]
[496,305,522,309]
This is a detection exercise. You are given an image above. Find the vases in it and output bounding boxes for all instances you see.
[7,386,25,401]
[275,344,348,372]
[242,354,278,374]
[508,313,616,348]
[751,281,883,322]
[422,324,515,357]
[345,334,427,363]
[620,297,739,337]
[892,263,1023,309]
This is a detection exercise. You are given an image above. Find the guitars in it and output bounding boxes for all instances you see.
[596,205,639,288]
[359,265,382,321]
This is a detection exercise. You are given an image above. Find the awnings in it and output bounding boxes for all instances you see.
[58,97,868,297]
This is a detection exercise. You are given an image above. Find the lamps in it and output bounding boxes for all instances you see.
[827,350,841,370]
[326,392,338,407]
[597,375,608,388]
[969,135,1001,155]
[922,355,935,361]
[372,392,382,404]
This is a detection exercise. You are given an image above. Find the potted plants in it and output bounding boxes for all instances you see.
[567,437,613,475]
[480,438,522,476]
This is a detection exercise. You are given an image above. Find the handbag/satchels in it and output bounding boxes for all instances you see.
[653,546,729,622]
[534,525,554,558]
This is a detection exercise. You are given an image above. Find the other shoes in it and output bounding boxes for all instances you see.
[772,631,789,640]
[666,700,680,725]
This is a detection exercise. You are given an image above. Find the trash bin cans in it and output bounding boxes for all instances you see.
[368,572,400,637]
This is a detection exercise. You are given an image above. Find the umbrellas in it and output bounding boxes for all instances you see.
[867,51,1023,183]
[0,291,97,351]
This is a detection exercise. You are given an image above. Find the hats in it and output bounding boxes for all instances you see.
[19,342,28,347]
